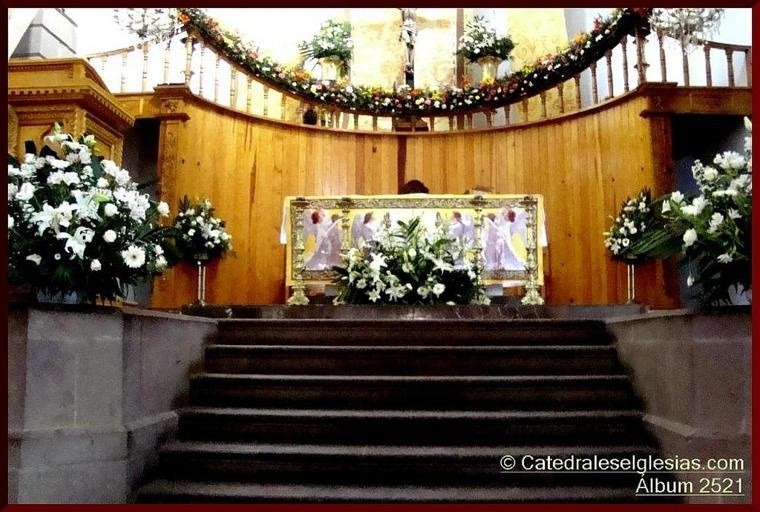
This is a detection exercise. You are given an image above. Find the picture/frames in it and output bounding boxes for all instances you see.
[284,193,545,288]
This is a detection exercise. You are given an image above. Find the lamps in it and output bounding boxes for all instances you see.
[112,7,179,50]
[648,7,726,55]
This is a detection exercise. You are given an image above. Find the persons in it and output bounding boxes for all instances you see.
[399,9,418,75]
[298,205,529,271]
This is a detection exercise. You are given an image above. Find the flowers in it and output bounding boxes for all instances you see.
[175,8,630,116]
[329,214,478,306]
[7,121,234,307]
[602,116,752,306]
